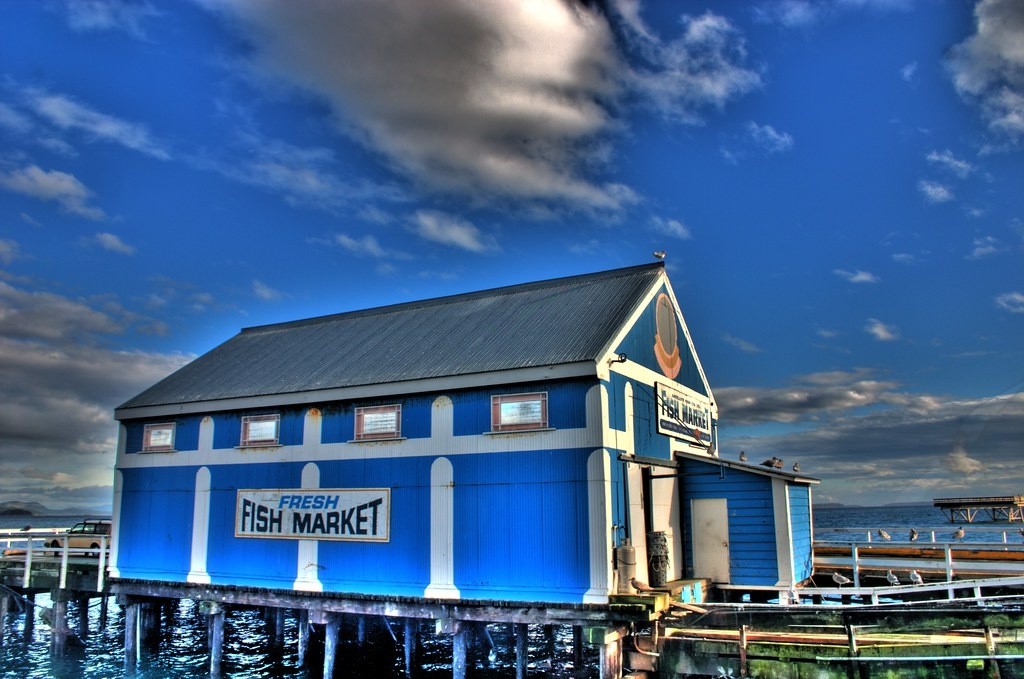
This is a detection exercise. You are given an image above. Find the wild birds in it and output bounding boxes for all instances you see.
[628,577,654,594]
[832,572,854,589]
[908,529,918,541]
[909,569,924,585]
[887,569,901,586]
[1017,527,1024,536]
[788,582,800,604]
[878,529,891,541]
[739,451,747,462]
[760,457,784,471]
[951,526,965,544]
[20,523,33,531]
[793,462,801,475]
[653,250,667,260]
[707,442,715,457]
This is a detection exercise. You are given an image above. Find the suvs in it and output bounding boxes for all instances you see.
[45,519,112,558]
